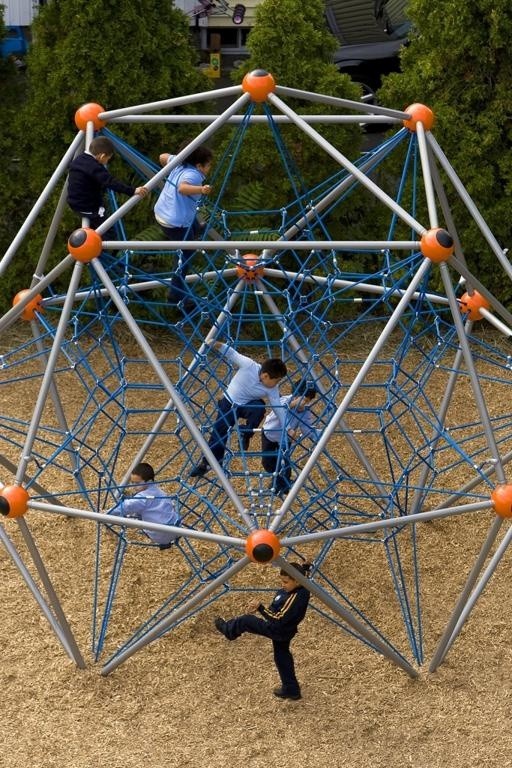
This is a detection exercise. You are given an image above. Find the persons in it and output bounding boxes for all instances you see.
[190,339,300,477]
[98,463,180,545]
[213,562,310,699]
[261,379,326,494]
[67,136,149,262]
[153,146,213,307]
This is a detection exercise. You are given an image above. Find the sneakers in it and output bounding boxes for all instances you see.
[215,617,236,641]
[273,689,301,700]
[188,456,211,477]
[268,477,290,494]
[237,424,249,449]
